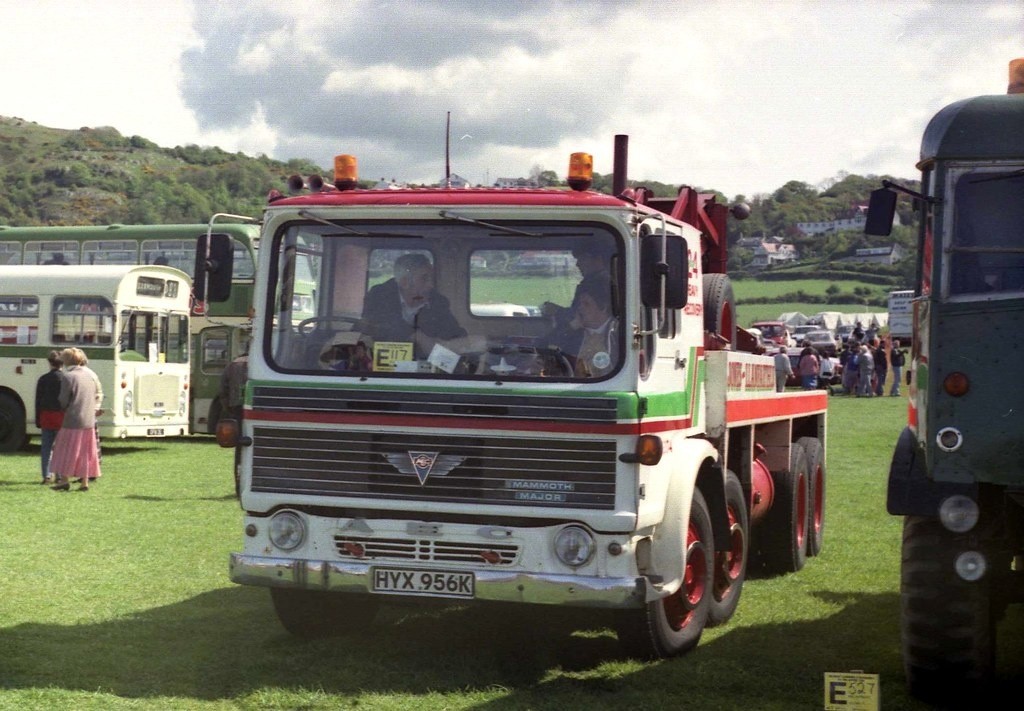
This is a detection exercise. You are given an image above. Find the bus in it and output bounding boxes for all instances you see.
[0,265,195,453]
[1,224,261,435]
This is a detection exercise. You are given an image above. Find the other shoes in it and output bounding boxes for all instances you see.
[76,487,88,491]
[55,478,62,483]
[42,478,51,486]
[51,483,70,491]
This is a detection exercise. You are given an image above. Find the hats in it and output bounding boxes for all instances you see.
[779,345,788,352]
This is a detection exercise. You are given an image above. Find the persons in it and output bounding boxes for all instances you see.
[35,348,103,491]
[220,340,249,496]
[841,322,909,397]
[362,252,470,376]
[540,241,620,377]
[775,341,834,397]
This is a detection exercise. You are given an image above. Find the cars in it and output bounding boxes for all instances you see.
[749,321,854,384]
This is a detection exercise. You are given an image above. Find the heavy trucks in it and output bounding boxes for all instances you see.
[862,59,1023,710]
[228,151,830,661]
